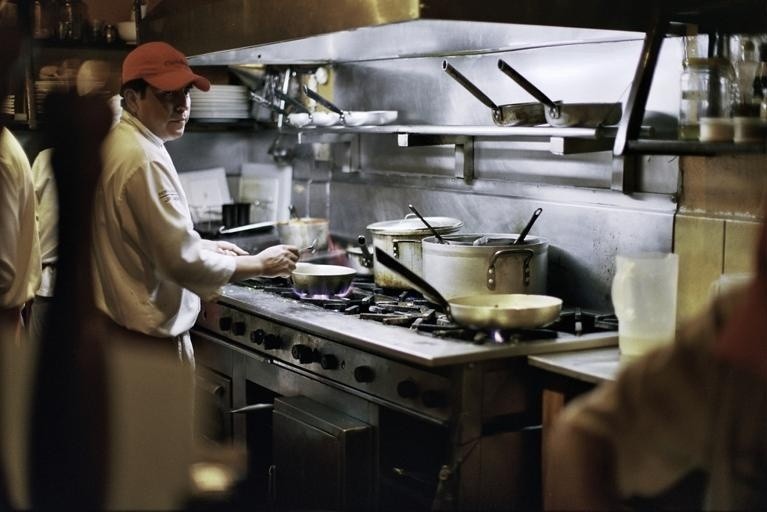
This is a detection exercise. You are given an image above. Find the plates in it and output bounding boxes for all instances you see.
[188,81,248,123]
[2,95,44,118]
[75,78,112,122]
[35,69,75,120]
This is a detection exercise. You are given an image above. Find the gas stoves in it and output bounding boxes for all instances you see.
[199,267,620,428]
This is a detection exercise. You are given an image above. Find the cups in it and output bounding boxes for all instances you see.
[612,252,678,356]
[221,203,249,227]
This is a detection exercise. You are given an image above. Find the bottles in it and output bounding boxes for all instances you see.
[675,32,767,146]
[49,1,115,43]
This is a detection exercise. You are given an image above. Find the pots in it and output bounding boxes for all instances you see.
[276,217,331,254]
[439,55,622,128]
[249,82,398,126]
[346,237,373,276]
[367,213,565,329]
[287,263,357,298]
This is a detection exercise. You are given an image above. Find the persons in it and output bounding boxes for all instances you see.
[28,145,61,353]
[0,122,43,344]
[543,223,767,509]
[93,39,304,511]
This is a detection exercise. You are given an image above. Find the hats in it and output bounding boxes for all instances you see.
[122,41,211,92]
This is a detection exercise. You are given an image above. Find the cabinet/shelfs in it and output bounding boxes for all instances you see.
[22,0,137,130]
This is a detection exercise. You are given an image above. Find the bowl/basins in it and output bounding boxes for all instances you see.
[116,22,138,44]
